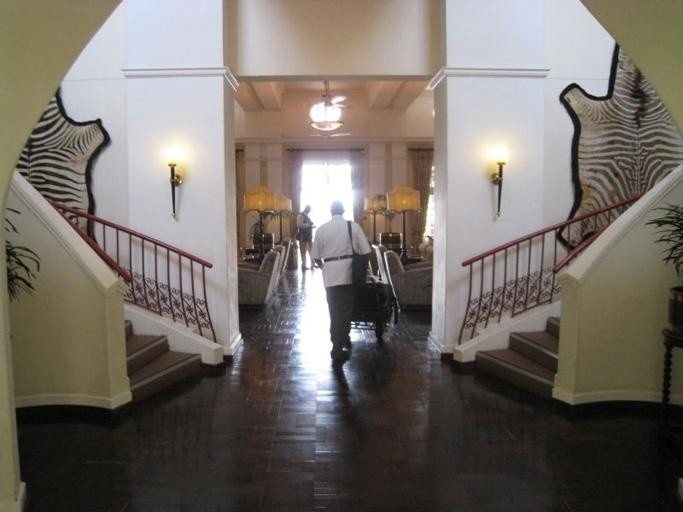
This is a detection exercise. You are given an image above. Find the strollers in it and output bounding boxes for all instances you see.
[342,242,404,348]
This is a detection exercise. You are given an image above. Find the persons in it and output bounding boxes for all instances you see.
[309,200,373,361]
[295,205,319,271]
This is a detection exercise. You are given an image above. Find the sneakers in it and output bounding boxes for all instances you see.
[302,265,318,270]
[331,350,349,359]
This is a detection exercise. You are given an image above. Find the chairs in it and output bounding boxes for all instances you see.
[368,244,432,311]
[237,238,293,310]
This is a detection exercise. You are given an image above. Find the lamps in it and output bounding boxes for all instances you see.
[309,80,347,134]
[242,185,292,263]
[364,186,420,261]
[490,146,506,218]
[166,140,181,218]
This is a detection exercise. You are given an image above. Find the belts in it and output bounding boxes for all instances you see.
[324,255,355,263]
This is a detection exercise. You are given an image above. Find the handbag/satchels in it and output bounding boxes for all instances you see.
[353,255,370,288]
